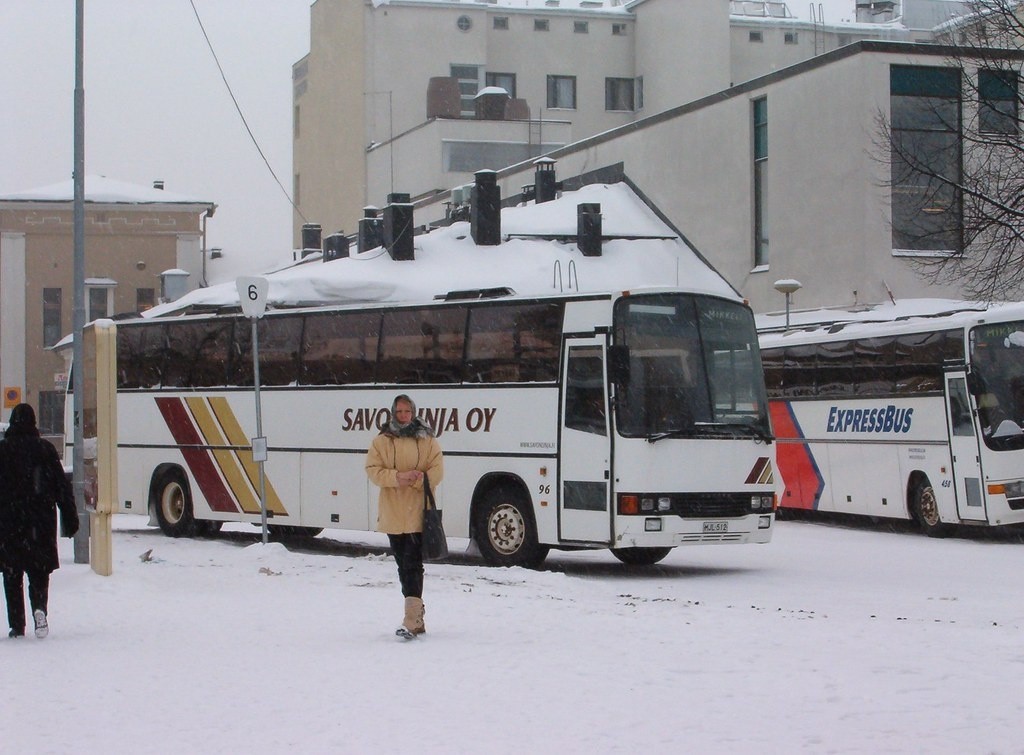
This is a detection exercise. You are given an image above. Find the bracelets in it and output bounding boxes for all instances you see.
[410,481,415,487]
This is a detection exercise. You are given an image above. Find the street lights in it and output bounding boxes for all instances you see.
[775,279,799,330]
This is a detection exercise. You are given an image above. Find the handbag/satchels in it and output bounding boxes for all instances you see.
[28,482,56,517]
[422,510,449,562]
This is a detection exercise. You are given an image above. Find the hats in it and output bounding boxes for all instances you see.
[10,402,37,424]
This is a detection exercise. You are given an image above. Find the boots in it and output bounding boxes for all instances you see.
[396,596,426,639]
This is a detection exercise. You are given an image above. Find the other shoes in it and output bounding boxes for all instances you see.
[8,629,25,638]
[33,606,49,638]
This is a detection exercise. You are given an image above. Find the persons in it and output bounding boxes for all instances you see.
[365,394,443,639]
[0,404,78,639]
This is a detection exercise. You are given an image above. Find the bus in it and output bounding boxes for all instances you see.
[741,280,1024,540]
[55,285,784,572]
